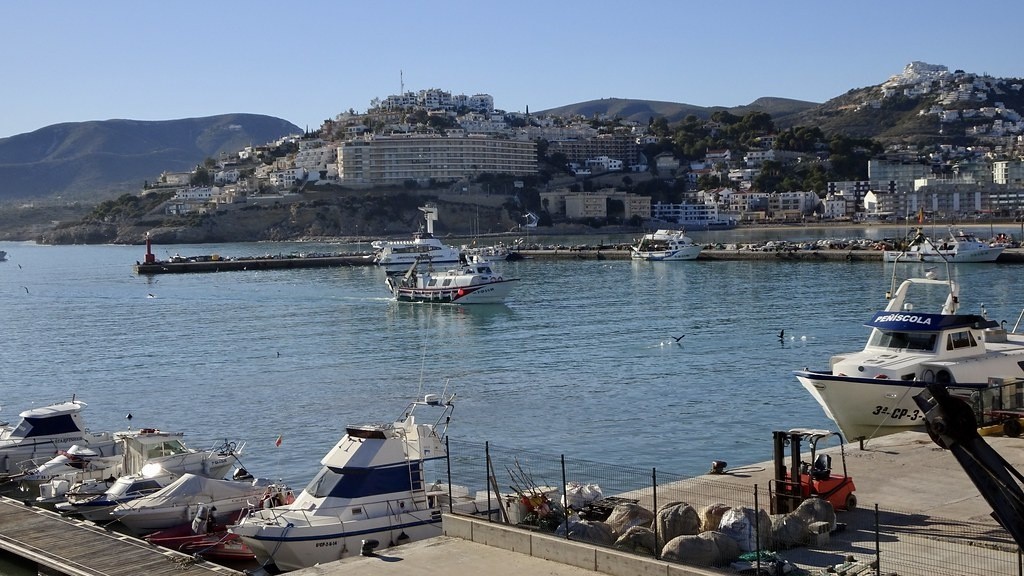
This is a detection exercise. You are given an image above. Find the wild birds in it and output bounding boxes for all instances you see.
[671,335,686,342]
[776,329,785,338]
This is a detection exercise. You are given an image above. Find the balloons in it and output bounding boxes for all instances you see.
[457,289,463,296]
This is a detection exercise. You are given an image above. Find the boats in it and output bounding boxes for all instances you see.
[110,471,286,534]
[883,227,1007,263]
[791,233,1024,444]
[385,249,519,304]
[1,393,245,501]
[631,228,705,262]
[372,223,510,264]
[66,464,183,522]
[142,508,257,562]
[226,377,511,574]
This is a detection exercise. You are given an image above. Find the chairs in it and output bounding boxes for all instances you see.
[808,454,832,479]
